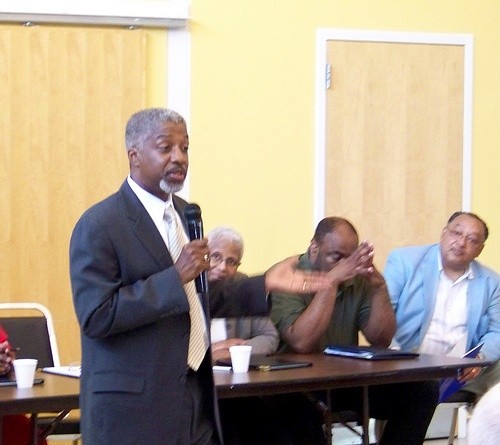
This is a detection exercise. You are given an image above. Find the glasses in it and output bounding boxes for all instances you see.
[210,253,241,266]
[447,226,482,248]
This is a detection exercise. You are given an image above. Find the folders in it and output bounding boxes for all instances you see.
[324,346,419,360]
[214,354,314,371]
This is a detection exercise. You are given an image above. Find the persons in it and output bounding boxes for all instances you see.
[204,226,279,365]
[382,211,500,445]
[69,109,338,445]
[0,323,48,445]
[270,216,439,445]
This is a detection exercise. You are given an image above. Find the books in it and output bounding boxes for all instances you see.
[323,346,420,360]
[215,354,313,372]
[42,366,81,378]
[440,376,465,404]
[0,373,44,387]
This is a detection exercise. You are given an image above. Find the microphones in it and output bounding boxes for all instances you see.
[184,203,206,294]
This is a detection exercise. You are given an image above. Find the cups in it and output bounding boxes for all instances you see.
[13,358,38,390]
[229,345,253,374]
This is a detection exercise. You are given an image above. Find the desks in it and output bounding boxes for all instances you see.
[0,352,494,445]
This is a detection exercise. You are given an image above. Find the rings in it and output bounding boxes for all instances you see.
[303,282,306,288]
[204,254,209,261]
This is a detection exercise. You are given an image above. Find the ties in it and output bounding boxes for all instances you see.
[164,206,206,372]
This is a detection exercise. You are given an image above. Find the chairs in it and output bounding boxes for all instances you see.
[0,303,60,368]
